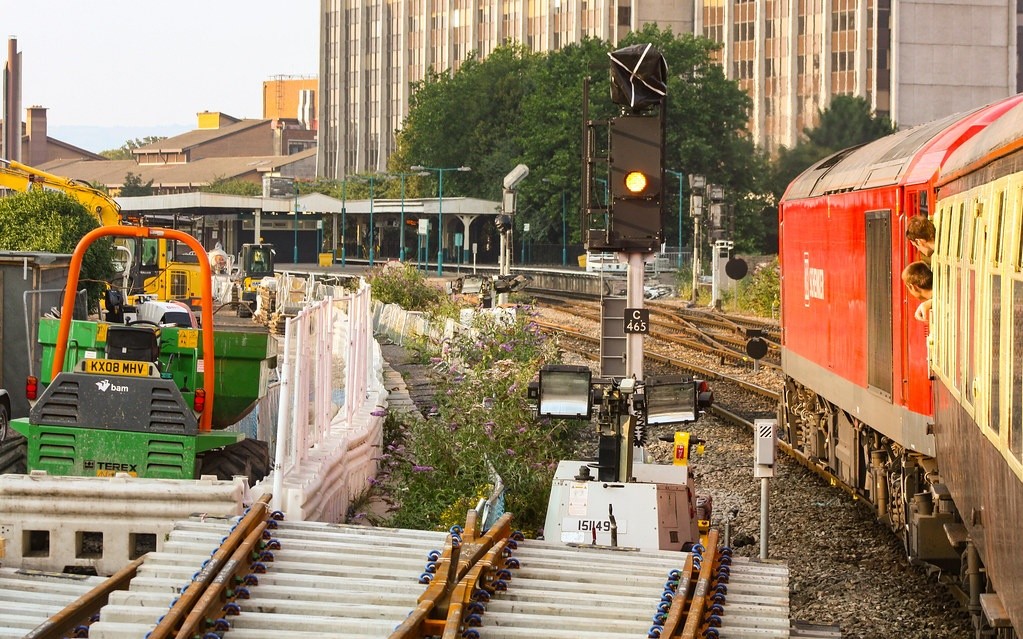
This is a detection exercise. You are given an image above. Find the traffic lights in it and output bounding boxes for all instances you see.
[611,119,661,198]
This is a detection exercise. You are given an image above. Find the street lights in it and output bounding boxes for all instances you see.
[410,165,472,277]
[542,177,566,267]
[345,174,398,265]
[293,181,321,264]
[374,170,431,263]
[318,178,368,267]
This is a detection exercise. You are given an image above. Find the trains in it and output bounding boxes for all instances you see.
[777,93,1023,639]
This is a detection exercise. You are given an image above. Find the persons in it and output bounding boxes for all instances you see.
[146,246,156,265]
[902,261,933,321]
[905,215,936,257]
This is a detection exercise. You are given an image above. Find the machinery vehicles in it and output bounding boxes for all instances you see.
[0,155,277,489]
